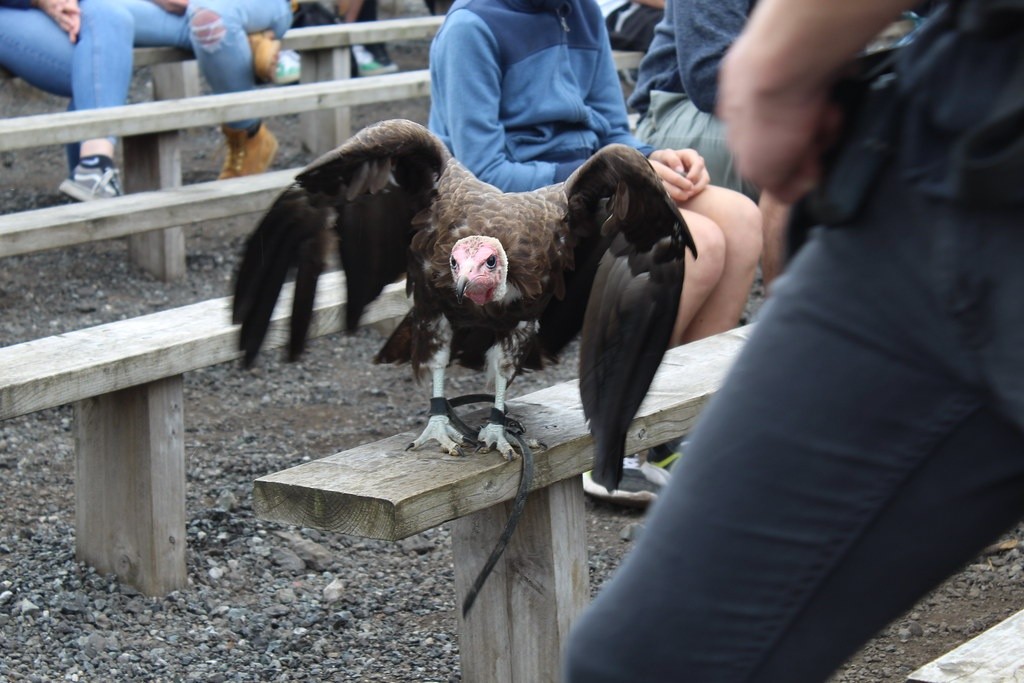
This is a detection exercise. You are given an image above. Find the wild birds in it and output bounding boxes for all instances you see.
[230,116,699,496]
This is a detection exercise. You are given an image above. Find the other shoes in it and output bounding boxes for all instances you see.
[352,46,398,77]
[219,125,278,181]
[249,30,280,73]
[275,50,303,84]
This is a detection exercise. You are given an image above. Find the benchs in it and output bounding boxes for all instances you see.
[0,0,1024,683]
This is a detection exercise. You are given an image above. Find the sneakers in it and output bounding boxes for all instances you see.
[59,154,122,202]
[582,437,688,499]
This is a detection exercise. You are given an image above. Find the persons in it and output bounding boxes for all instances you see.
[569,0,1024,683]
[0,0,399,203]
[429,0,918,505]
